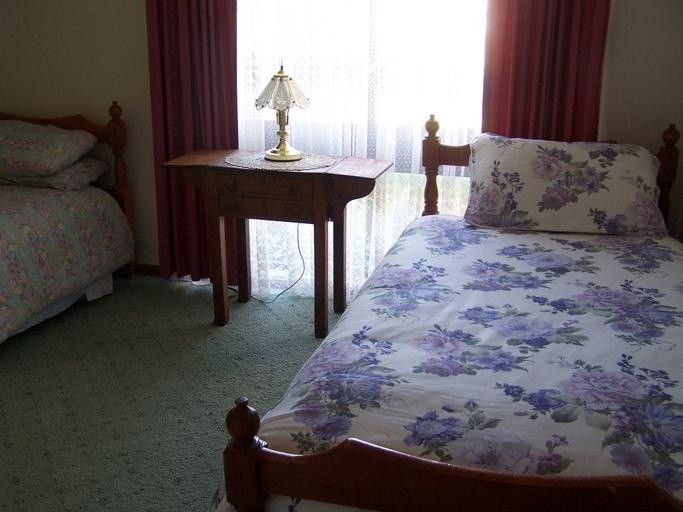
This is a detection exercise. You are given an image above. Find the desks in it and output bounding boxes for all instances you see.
[158,147,395,337]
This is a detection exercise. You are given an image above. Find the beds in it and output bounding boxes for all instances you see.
[0,99,136,340]
[203,115,683,509]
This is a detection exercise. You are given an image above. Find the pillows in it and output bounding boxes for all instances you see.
[0,119,113,191]
[463,131,667,238]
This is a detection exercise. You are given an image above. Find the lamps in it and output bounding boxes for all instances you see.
[252,62,312,161]
